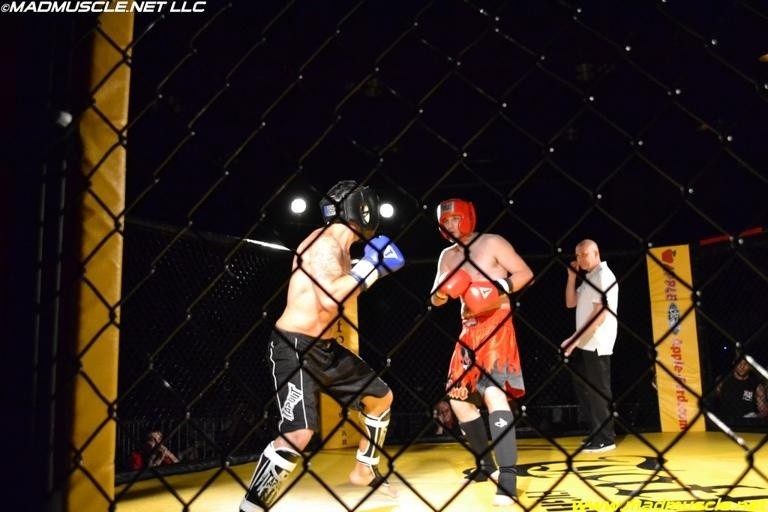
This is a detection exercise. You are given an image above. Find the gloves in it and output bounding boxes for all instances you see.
[349,236,406,291]
[437,269,510,314]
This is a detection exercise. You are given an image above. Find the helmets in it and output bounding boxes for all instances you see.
[319,180,380,229]
[436,198,476,240]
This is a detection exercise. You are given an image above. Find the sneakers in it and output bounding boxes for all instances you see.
[580,434,616,454]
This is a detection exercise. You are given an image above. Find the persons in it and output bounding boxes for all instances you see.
[425,197,537,507]
[429,346,767,432]
[118,430,251,475]
[235,176,406,512]
[560,240,622,453]
